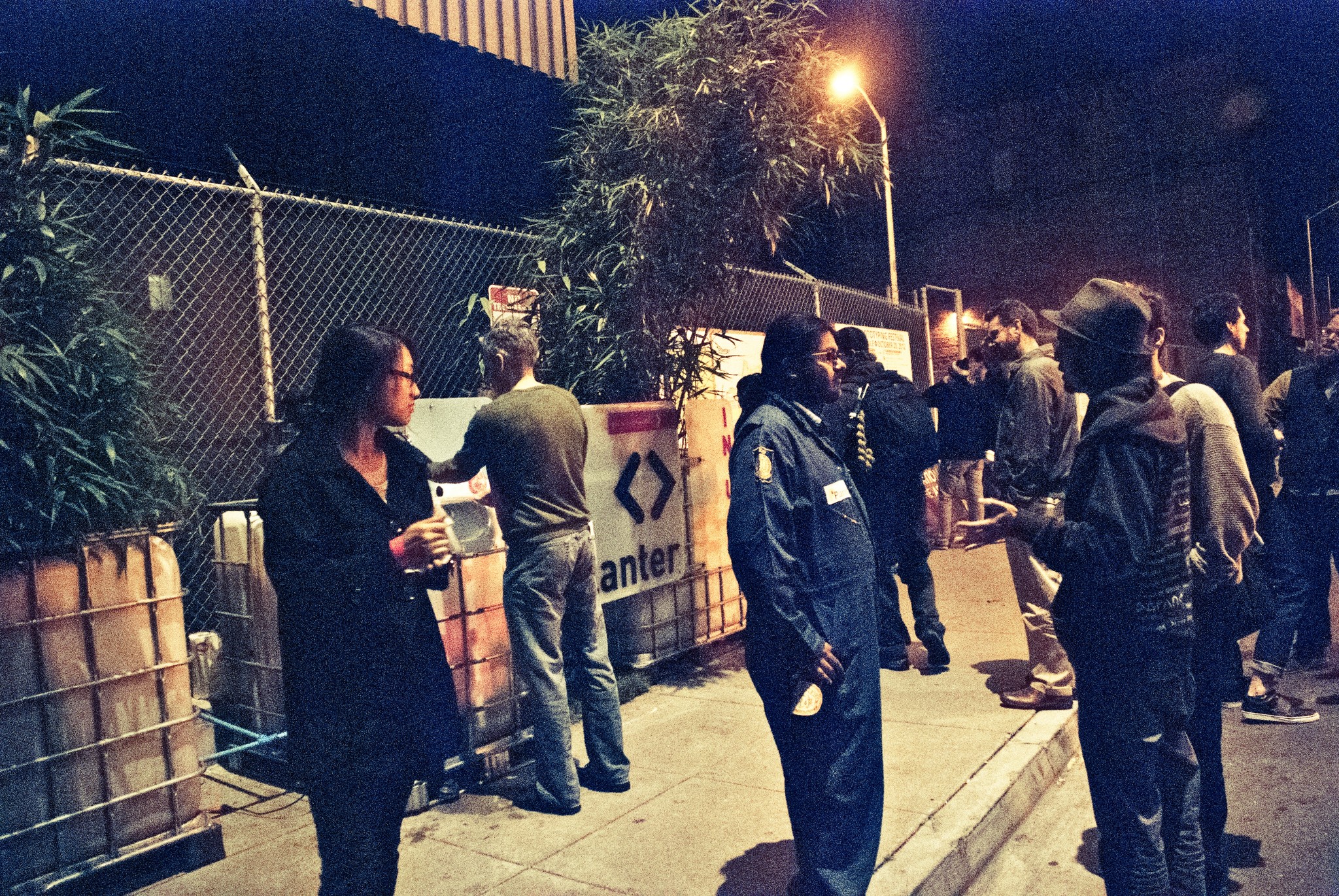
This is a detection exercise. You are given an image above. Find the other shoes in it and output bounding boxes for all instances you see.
[879,647,911,671]
[514,784,580,815]
[1282,647,1333,674]
[930,539,948,549]
[953,535,982,548]
[920,629,951,665]
[575,767,631,793]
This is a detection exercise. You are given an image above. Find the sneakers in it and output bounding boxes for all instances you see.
[1240,692,1319,723]
[1221,686,1246,708]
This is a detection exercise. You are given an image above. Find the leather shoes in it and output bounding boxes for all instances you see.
[1001,683,1073,710]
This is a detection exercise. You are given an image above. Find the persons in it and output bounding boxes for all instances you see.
[425,317,631,817]
[1239,305,1339,724]
[922,345,1002,549]
[985,297,1077,710]
[821,328,950,671]
[1124,281,1259,896]
[257,325,470,896]
[727,314,884,896]
[1192,291,1277,710]
[953,276,1203,895]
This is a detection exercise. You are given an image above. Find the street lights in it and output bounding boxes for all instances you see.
[830,68,902,304]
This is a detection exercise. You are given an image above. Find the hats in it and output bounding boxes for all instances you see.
[949,357,971,381]
[1040,277,1155,358]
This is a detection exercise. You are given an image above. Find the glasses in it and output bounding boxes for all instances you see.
[387,366,418,389]
[810,347,846,362]
[1321,326,1339,339]
[983,315,1012,347]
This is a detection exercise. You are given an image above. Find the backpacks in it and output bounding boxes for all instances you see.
[844,374,941,475]
